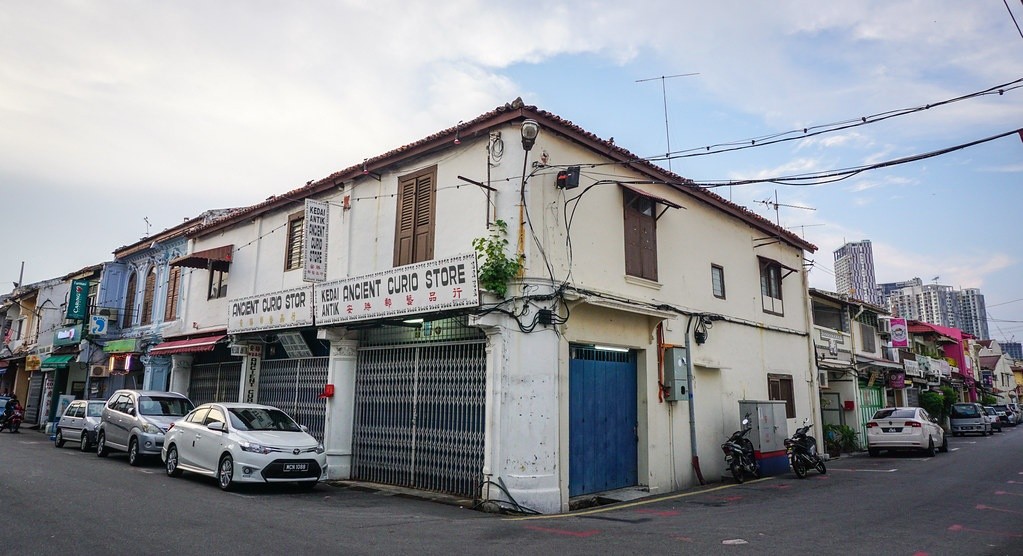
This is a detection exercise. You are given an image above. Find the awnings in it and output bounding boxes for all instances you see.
[41,354,76,368]
[169,245,233,273]
[149,335,228,355]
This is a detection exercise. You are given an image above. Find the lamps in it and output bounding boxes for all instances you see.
[521,118,541,201]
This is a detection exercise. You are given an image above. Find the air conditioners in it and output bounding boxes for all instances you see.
[90,365,110,378]
[878,317,894,333]
[96,307,117,320]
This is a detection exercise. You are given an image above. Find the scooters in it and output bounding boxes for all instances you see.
[0,403,31,433]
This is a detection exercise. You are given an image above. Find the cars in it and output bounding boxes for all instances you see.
[0,396,11,417]
[983,406,1003,433]
[991,403,1023,427]
[161,401,329,493]
[865,407,948,457]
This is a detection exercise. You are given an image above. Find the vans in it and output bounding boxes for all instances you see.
[950,402,993,438]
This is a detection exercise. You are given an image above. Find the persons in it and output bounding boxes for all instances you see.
[2,394,21,427]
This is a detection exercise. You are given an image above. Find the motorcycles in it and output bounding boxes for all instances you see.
[720,418,761,483]
[783,418,827,480]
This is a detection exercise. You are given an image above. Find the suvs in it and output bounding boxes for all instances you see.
[54,399,112,452]
[96,388,198,465]
